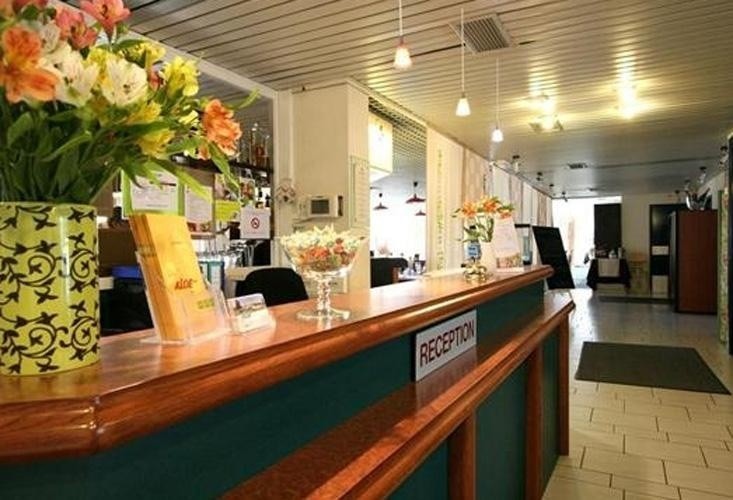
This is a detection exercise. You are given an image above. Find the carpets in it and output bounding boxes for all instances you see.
[574,342,730,394]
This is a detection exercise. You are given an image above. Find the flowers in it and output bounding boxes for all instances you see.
[450,194,516,242]
[0,0,262,204]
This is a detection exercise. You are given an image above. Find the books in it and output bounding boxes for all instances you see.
[128,212,217,341]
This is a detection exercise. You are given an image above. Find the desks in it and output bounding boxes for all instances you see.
[592,257,627,294]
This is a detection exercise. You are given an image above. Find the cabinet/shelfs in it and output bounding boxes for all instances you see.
[667,210,717,316]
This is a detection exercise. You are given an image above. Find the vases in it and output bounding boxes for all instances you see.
[0,201,101,376]
[479,242,497,274]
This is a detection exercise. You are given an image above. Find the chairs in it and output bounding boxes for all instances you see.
[96,287,154,337]
[236,268,308,307]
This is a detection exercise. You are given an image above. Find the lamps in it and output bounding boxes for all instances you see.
[368,112,394,184]
[492,54,503,142]
[393,0,412,70]
[456,7,470,117]
[416,210,425,216]
[374,193,387,210]
[406,181,425,203]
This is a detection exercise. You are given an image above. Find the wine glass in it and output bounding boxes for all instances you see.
[274,235,365,319]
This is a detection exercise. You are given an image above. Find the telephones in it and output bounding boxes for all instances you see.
[297,193,338,220]
